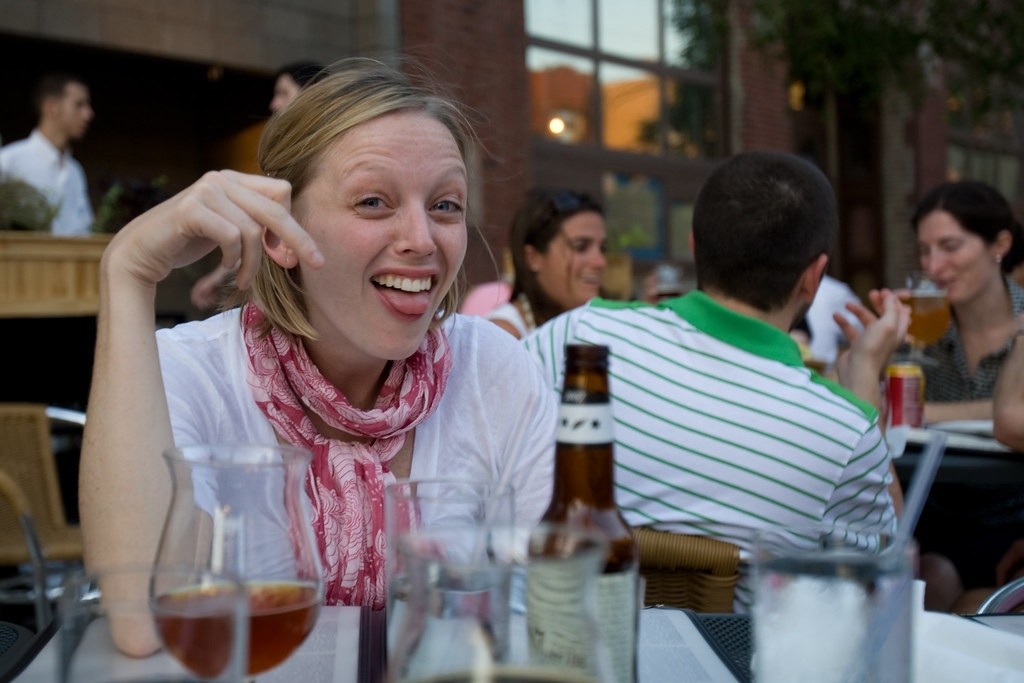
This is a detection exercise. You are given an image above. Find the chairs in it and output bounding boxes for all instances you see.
[632,532,880,615]
[0,404,85,630]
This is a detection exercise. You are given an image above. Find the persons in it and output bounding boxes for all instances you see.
[456,140,1022,613]
[79,52,559,657]
[192,61,327,312]
[0,71,96,237]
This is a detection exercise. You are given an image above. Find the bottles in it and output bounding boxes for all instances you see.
[522,341,645,681]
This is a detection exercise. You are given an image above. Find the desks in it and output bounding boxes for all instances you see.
[71,608,1024,683]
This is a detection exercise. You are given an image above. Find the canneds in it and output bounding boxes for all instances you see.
[884,364,926,429]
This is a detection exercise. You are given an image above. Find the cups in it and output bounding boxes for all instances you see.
[149,441,325,682]
[748,527,917,682]
[385,478,516,682]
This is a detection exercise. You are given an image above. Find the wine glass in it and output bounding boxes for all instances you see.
[891,273,952,368]
